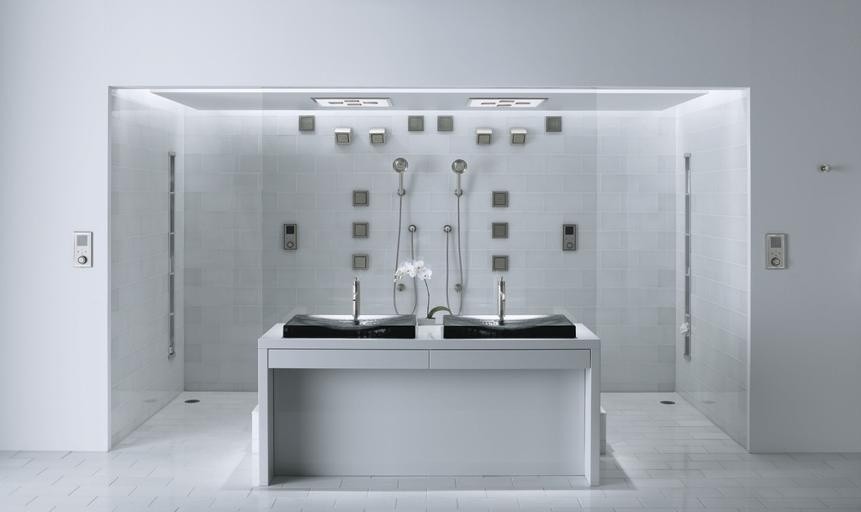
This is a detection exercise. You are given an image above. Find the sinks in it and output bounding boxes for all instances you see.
[442,313,576,339]
[283,314,419,338]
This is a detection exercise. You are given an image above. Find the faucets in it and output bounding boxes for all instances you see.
[352,276,361,326]
[497,276,506,325]
